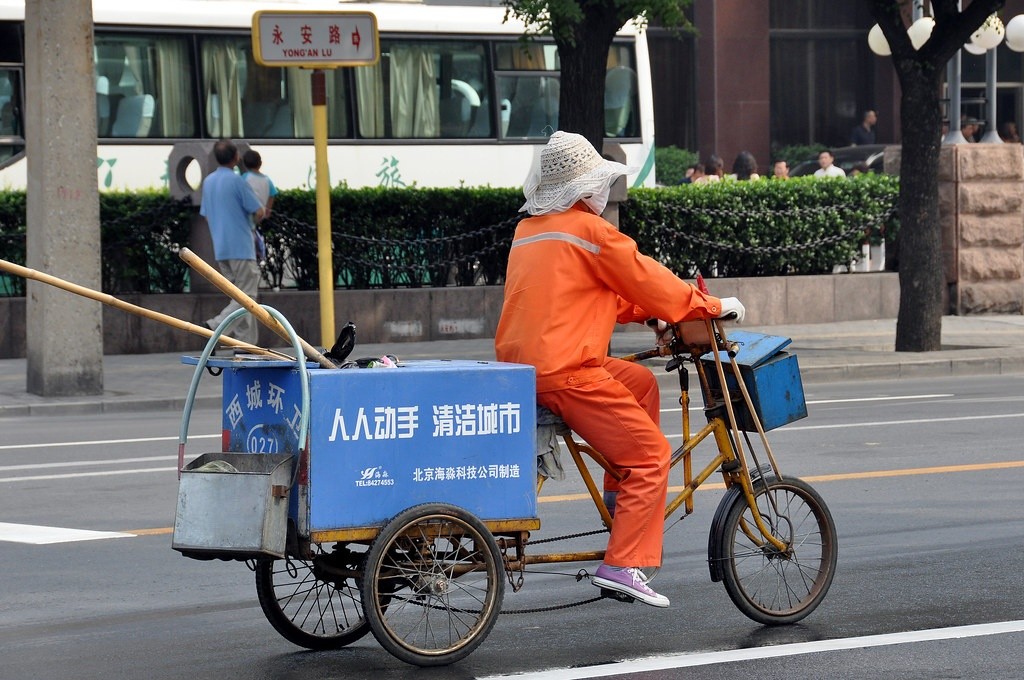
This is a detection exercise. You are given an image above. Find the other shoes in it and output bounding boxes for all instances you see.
[199,321,216,358]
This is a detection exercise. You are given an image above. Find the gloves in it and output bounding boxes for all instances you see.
[645,317,672,346]
[715,296,747,325]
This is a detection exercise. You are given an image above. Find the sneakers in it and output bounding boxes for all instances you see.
[591,562,670,608]
[600,506,614,523]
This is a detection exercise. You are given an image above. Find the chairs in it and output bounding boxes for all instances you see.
[96,75,156,137]
[209,95,294,138]
[448,66,636,136]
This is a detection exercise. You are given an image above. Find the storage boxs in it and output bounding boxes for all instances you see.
[702,329,808,432]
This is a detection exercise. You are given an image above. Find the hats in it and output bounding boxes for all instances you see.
[516,130,629,217]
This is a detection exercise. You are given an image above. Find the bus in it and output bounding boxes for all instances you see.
[0,4,659,200]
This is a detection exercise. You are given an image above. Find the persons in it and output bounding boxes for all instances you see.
[851,110,878,146]
[495,131,744,608]
[813,151,843,178]
[774,161,789,179]
[242,150,278,266]
[940,113,978,143]
[198,139,263,355]
[678,150,759,183]
[1004,122,1020,142]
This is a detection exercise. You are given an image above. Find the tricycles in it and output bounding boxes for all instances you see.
[192,319,845,670]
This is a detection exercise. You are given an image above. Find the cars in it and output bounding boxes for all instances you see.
[788,142,894,178]
